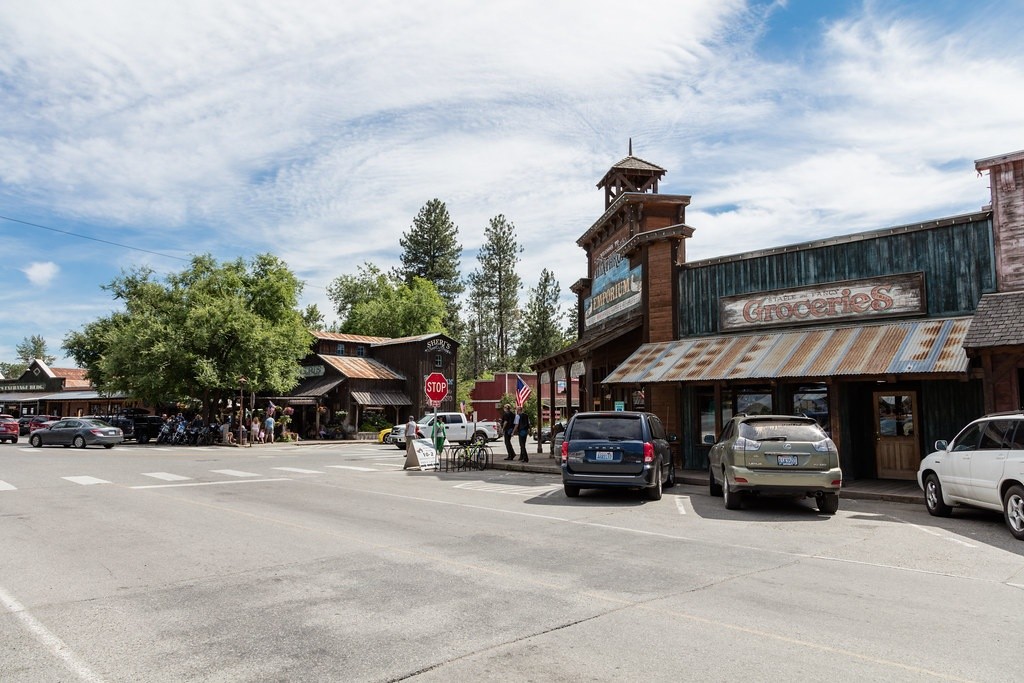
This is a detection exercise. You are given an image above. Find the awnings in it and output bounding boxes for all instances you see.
[603,316,977,383]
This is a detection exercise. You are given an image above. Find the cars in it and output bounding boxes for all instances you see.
[534,421,567,444]
[378,428,393,444]
[28,419,124,449]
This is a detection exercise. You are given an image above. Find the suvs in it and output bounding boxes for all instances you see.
[16,414,35,436]
[0,414,19,444]
[560,411,677,502]
[703,412,842,515]
[916,411,1024,540]
[29,415,60,433]
[108,408,164,444]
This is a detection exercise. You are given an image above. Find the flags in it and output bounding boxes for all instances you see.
[516,378,530,407]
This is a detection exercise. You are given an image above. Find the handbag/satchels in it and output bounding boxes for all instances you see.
[444,439,450,449]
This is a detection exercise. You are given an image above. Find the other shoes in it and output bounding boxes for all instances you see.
[519,457,523,461]
[522,459,529,463]
[404,455,407,457]
[504,458,513,461]
[271,442,273,444]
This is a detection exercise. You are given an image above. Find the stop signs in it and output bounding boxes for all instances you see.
[424,373,449,403]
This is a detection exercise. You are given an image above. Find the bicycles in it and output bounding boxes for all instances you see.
[453,435,489,471]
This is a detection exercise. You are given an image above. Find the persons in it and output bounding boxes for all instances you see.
[430,417,447,459]
[404,416,417,457]
[501,404,532,463]
[214,409,275,446]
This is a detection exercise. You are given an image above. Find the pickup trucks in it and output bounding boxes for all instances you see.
[391,412,499,450]
